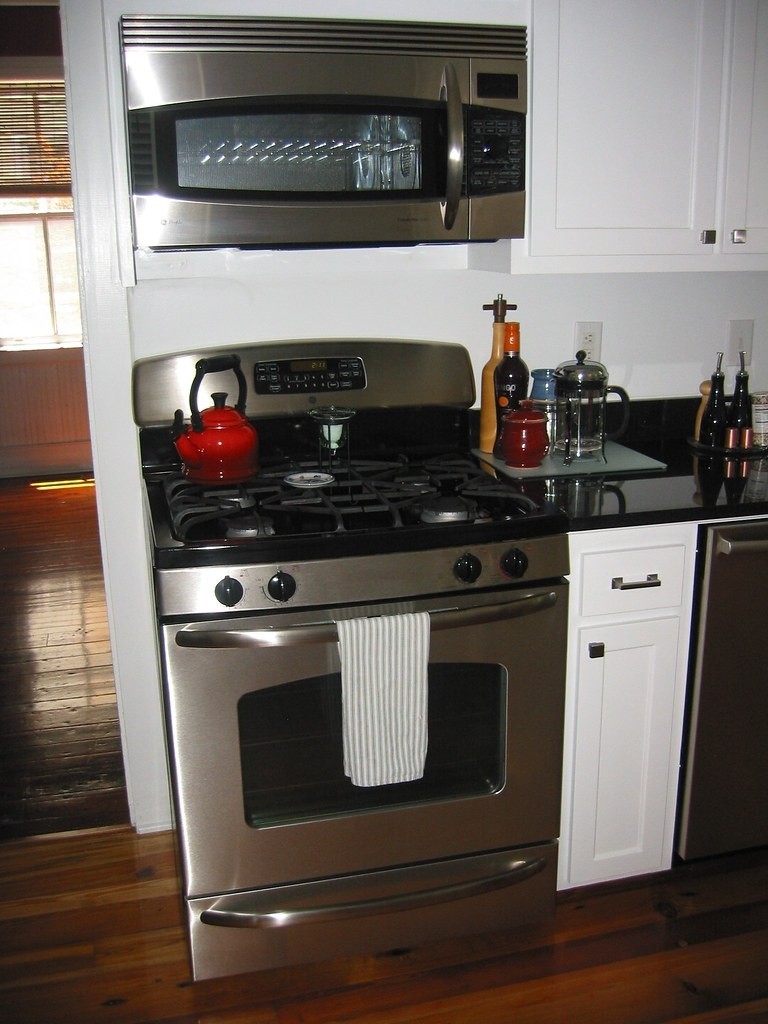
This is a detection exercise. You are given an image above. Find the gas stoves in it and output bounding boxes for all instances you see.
[132,338,570,617]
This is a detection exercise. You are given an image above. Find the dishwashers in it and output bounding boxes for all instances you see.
[677,518,767,862]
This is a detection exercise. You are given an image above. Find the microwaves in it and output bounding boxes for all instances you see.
[120,12,527,253]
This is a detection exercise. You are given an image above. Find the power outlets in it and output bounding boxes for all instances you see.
[573,321,602,364]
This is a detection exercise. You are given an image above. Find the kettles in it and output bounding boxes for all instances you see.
[168,354,258,487]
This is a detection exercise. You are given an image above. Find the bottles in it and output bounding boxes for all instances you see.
[693,456,702,505]
[695,380,712,442]
[699,352,726,447]
[697,458,724,507]
[724,457,750,504]
[725,351,753,449]
[530,370,557,454]
[492,322,529,458]
[479,293,517,454]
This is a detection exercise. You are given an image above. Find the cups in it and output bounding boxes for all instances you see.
[751,391,768,446]
[504,405,549,469]
[743,459,768,504]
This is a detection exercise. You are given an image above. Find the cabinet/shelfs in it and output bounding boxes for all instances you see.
[466,0,768,275]
[555,513,768,893]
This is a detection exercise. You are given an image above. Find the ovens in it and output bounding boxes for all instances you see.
[157,581,568,984]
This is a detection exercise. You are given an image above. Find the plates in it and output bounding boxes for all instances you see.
[284,473,335,490]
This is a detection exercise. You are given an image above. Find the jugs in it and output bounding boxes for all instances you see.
[550,350,631,461]
